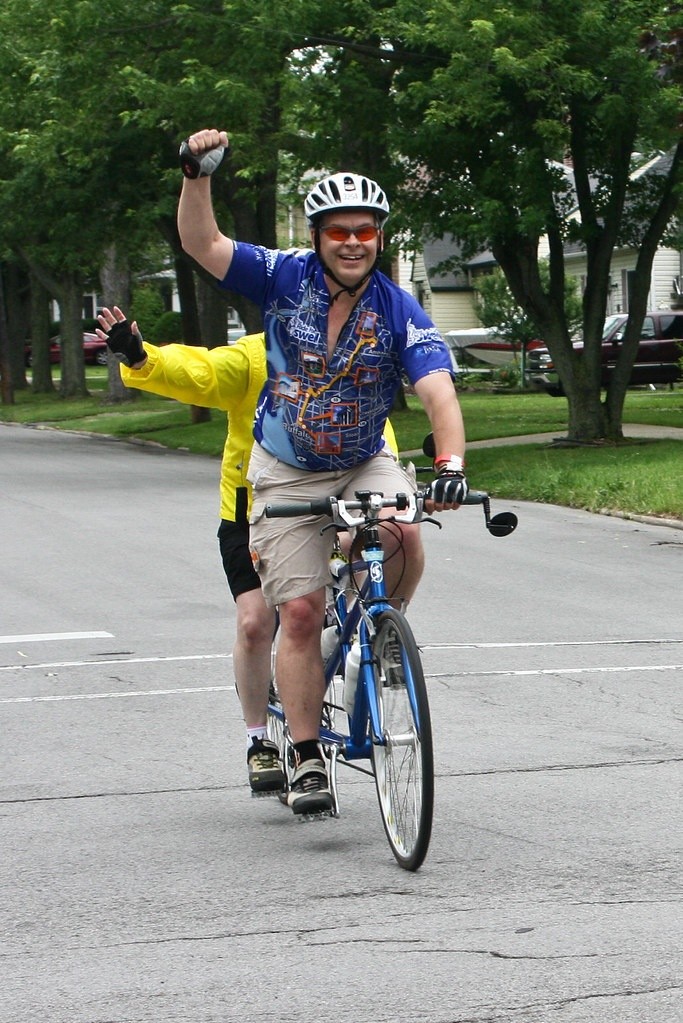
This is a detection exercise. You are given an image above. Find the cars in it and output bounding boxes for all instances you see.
[21,330,111,365]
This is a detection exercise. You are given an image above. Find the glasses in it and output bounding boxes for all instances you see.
[318,226,380,243]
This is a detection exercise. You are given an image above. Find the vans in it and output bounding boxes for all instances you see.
[523,307,683,398]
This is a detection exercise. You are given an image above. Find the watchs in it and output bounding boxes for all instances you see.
[436,462,464,473]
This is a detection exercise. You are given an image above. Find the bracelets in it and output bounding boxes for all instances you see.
[432,455,465,467]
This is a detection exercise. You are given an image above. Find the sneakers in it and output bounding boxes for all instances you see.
[286,759,337,815]
[374,628,407,684]
[247,735,285,791]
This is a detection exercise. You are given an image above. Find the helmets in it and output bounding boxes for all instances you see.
[302,173,390,229]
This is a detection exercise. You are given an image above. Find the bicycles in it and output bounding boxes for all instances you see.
[210,429,521,873]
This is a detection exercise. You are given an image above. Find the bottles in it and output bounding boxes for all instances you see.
[320,625,339,667]
[341,643,361,715]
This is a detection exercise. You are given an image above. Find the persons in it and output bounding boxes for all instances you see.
[94,306,399,794]
[175,123,466,820]
